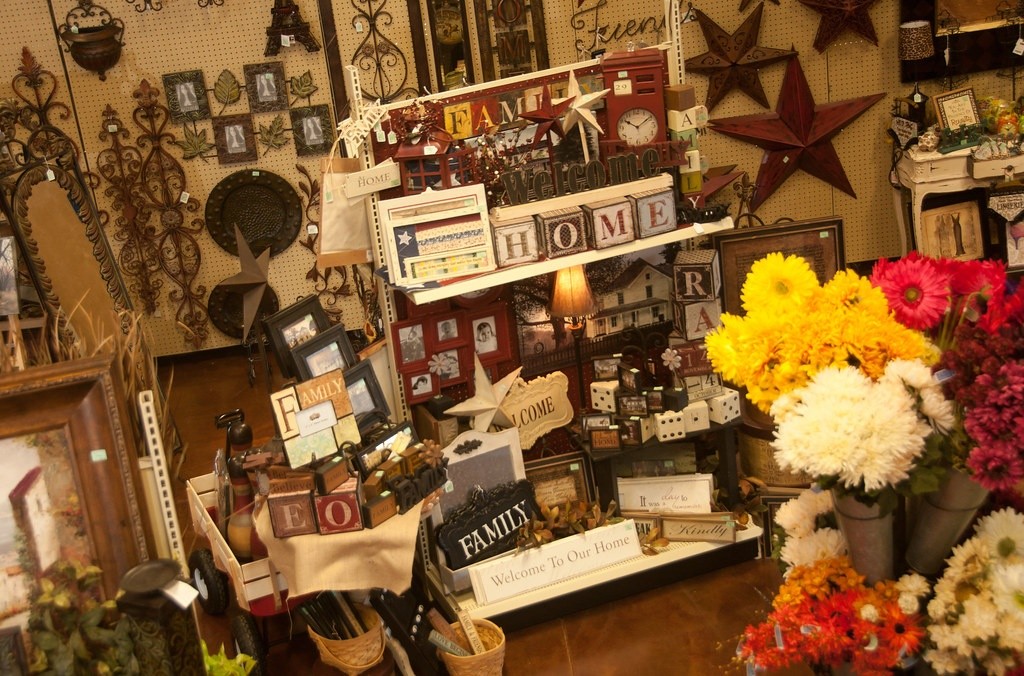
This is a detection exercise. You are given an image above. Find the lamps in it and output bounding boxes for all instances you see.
[547,264,601,435]
[897,20,934,122]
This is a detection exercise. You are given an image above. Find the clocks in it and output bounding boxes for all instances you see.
[997,117,1018,141]
[596,47,667,146]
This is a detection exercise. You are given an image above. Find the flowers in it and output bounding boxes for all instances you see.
[703,251,1024,676]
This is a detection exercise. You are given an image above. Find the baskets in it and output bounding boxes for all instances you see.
[307,607,385,676]
[436,618,505,676]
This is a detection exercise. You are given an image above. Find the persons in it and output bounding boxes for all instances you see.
[475,323,498,353]
[175,84,199,112]
[414,376,431,394]
[254,74,277,102]
[404,330,423,361]
[438,321,455,339]
[225,125,246,153]
[303,117,324,145]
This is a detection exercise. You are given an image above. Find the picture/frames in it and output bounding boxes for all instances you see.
[343,358,391,432]
[259,293,332,379]
[161,69,213,125]
[906,188,991,262]
[243,61,290,113]
[900,0,1024,84]
[289,104,334,157]
[389,300,514,407]
[288,323,359,386]
[211,113,258,166]
[1,352,161,667]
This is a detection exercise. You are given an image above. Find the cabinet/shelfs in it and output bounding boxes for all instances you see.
[892,141,1024,277]
[341,0,764,629]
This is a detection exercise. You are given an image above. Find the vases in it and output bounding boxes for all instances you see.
[903,461,989,573]
[829,484,894,586]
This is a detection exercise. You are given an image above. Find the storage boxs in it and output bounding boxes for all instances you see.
[186,471,291,611]
